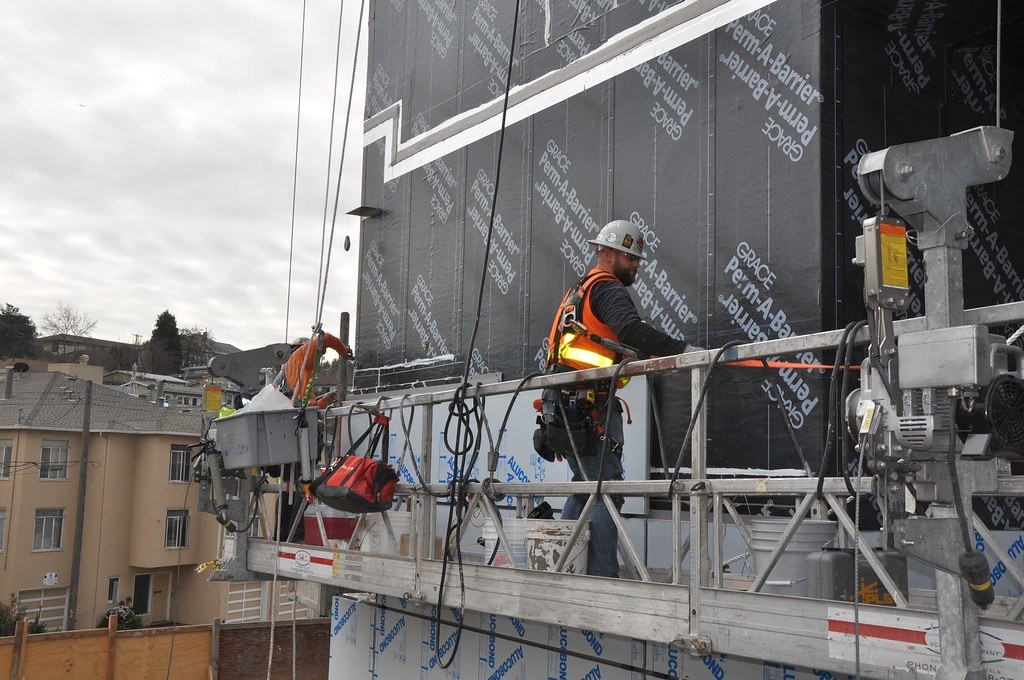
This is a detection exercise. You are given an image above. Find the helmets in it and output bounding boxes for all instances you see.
[291,337,310,346]
[586,219,646,259]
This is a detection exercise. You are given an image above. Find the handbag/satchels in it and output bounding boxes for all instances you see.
[308,414,400,513]
[547,415,595,457]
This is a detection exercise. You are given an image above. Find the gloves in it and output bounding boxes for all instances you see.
[685,344,705,353]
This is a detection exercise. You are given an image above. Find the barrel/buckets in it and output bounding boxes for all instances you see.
[361,509,412,556]
[527,518,592,575]
[484,515,525,567]
[748,521,839,597]
[859,545,909,607]
[805,543,855,598]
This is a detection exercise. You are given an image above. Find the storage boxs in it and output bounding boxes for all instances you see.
[305,504,360,545]
[211,405,319,469]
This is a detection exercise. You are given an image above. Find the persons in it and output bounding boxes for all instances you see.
[545,220,705,578]
[271,332,353,542]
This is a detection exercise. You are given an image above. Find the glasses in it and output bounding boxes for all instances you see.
[619,251,641,262]
